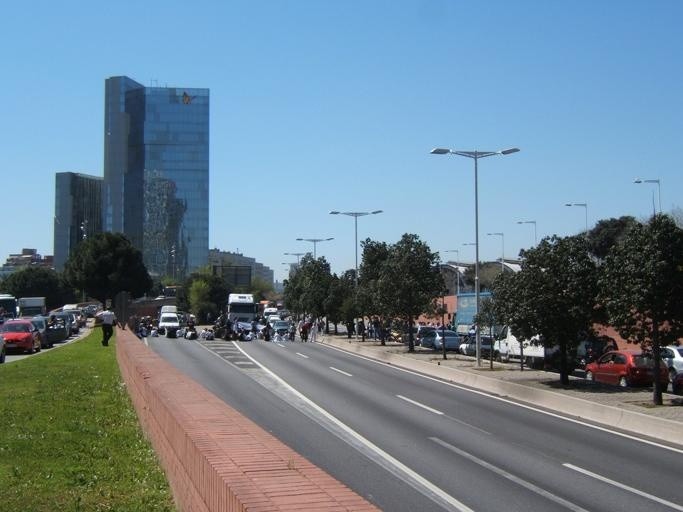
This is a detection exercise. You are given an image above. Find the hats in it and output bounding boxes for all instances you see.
[105,304,113,308]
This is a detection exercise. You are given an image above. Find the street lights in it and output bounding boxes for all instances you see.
[329,210,384,341]
[633,178,662,213]
[170,243,177,282]
[565,203,588,233]
[445,250,459,294]
[463,243,476,246]
[517,222,537,248]
[281,237,335,273]
[487,233,504,272]
[79,220,89,303]
[430,146,520,367]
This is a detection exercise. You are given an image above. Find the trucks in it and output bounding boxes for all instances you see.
[492,322,563,370]
[225,293,256,328]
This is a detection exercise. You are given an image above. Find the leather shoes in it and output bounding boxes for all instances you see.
[101,339,108,346]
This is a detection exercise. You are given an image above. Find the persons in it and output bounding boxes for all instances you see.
[419,321,456,332]
[96,307,122,346]
[48,315,57,348]
[213,311,325,343]
[168,312,214,340]
[132,313,165,339]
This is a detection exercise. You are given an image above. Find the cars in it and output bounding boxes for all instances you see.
[158,305,200,334]
[576,336,683,395]
[154,296,165,300]
[352,320,495,357]
[256,300,291,336]
[0,294,99,364]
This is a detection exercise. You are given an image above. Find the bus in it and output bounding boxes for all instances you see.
[162,286,183,301]
